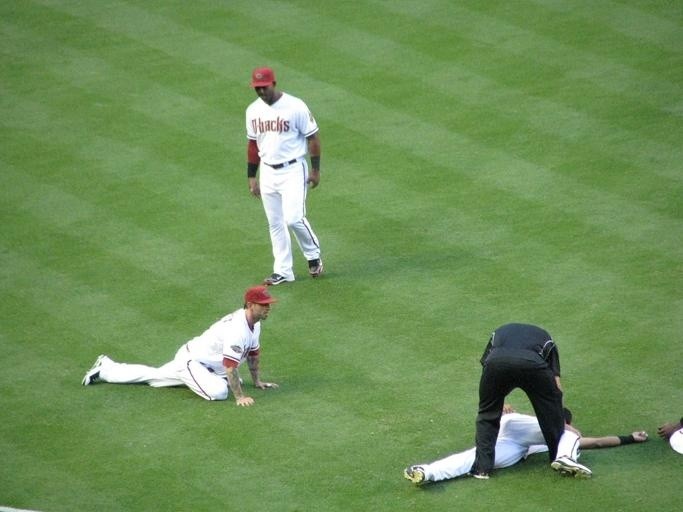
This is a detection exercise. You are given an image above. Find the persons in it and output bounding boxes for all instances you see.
[242,65,323,287]
[464,313,564,480]
[400,407,649,486]
[658,414,683,456]
[79,283,278,408]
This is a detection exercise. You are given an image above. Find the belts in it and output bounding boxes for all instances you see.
[187,343,228,382]
[264,159,296,169]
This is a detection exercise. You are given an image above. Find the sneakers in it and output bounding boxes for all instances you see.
[403,466,425,485]
[466,471,492,480]
[263,273,287,285]
[307,256,324,277]
[81,354,107,386]
[550,454,593,480]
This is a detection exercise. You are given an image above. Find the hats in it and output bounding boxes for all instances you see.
[250,68,275,88]
[244,286,277,305]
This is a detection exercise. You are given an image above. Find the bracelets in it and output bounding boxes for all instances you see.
[310,156,319,171]
[246,163,256,179]
[618,433,634,445]
